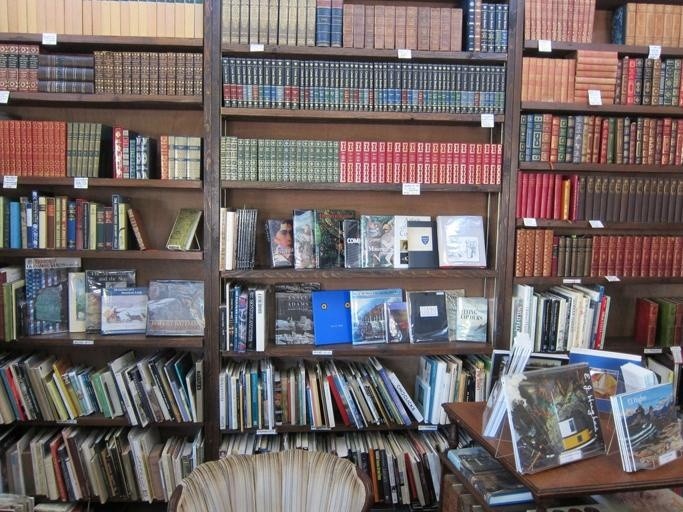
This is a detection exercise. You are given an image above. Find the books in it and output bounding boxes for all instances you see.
[220,1,509,512]
[510,0,683,512]
[1,0,204,512]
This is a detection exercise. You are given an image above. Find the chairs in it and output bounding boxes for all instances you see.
[165,447,373,512]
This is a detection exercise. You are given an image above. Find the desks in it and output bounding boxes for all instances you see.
[442,401,682,512]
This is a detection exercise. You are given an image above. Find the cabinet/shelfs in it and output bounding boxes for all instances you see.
[506,0,683,377]
[0,0,212,512]
[212,0,515,512]
